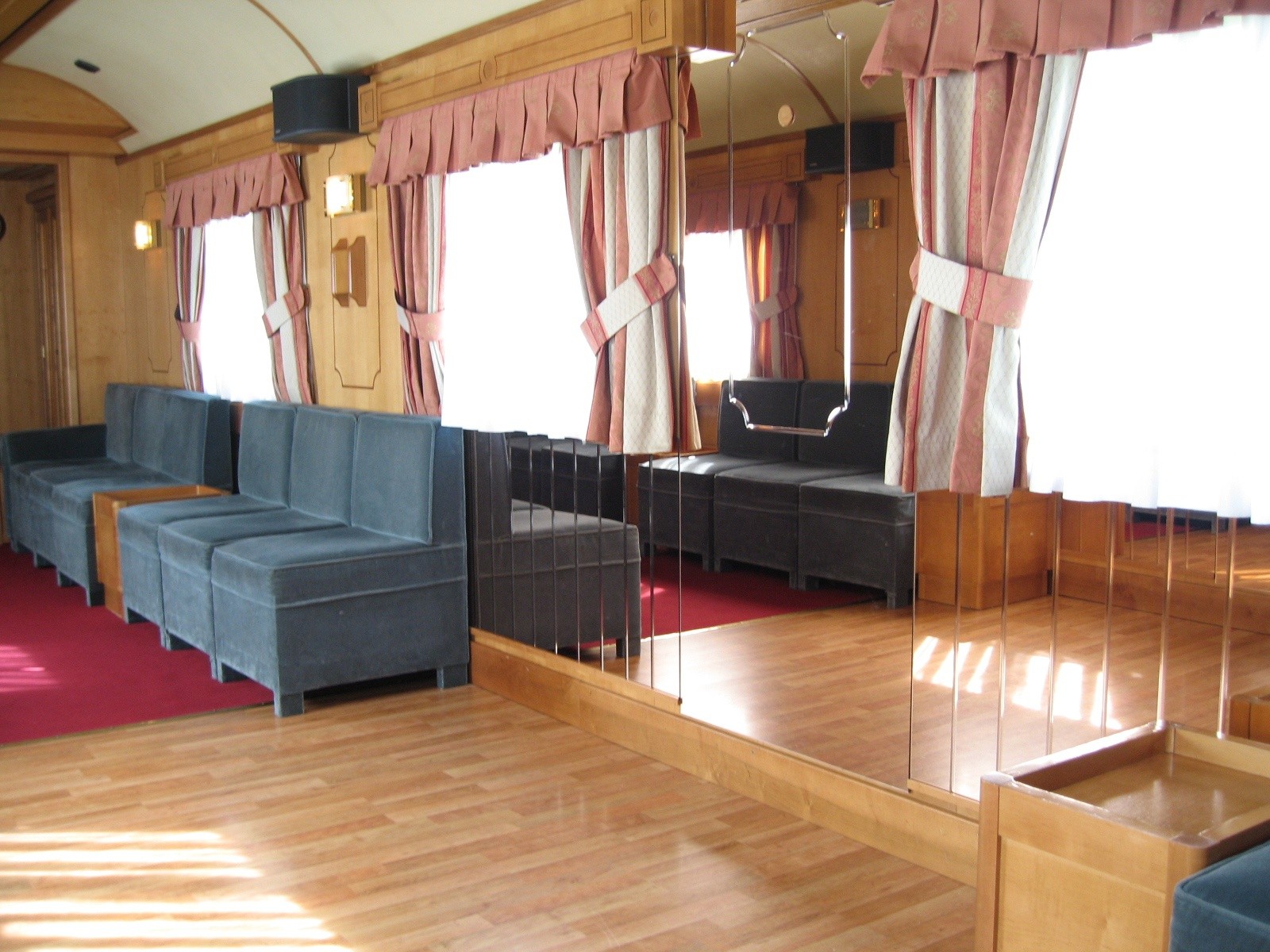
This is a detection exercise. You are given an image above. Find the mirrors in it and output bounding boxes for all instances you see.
[462,0,1270,804]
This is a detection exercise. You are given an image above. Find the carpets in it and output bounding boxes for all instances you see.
[0,541,274,745]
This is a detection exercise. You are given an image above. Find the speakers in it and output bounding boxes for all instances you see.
[805,120,895,173]
[271,72,370,145]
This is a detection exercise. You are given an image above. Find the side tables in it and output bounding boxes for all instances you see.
[976,719,1270,952]
[93,484,232,624]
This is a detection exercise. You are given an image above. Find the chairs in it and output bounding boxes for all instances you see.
[1171,848,1270,952]
[471,377,916,656]
[0,383,470,718]
